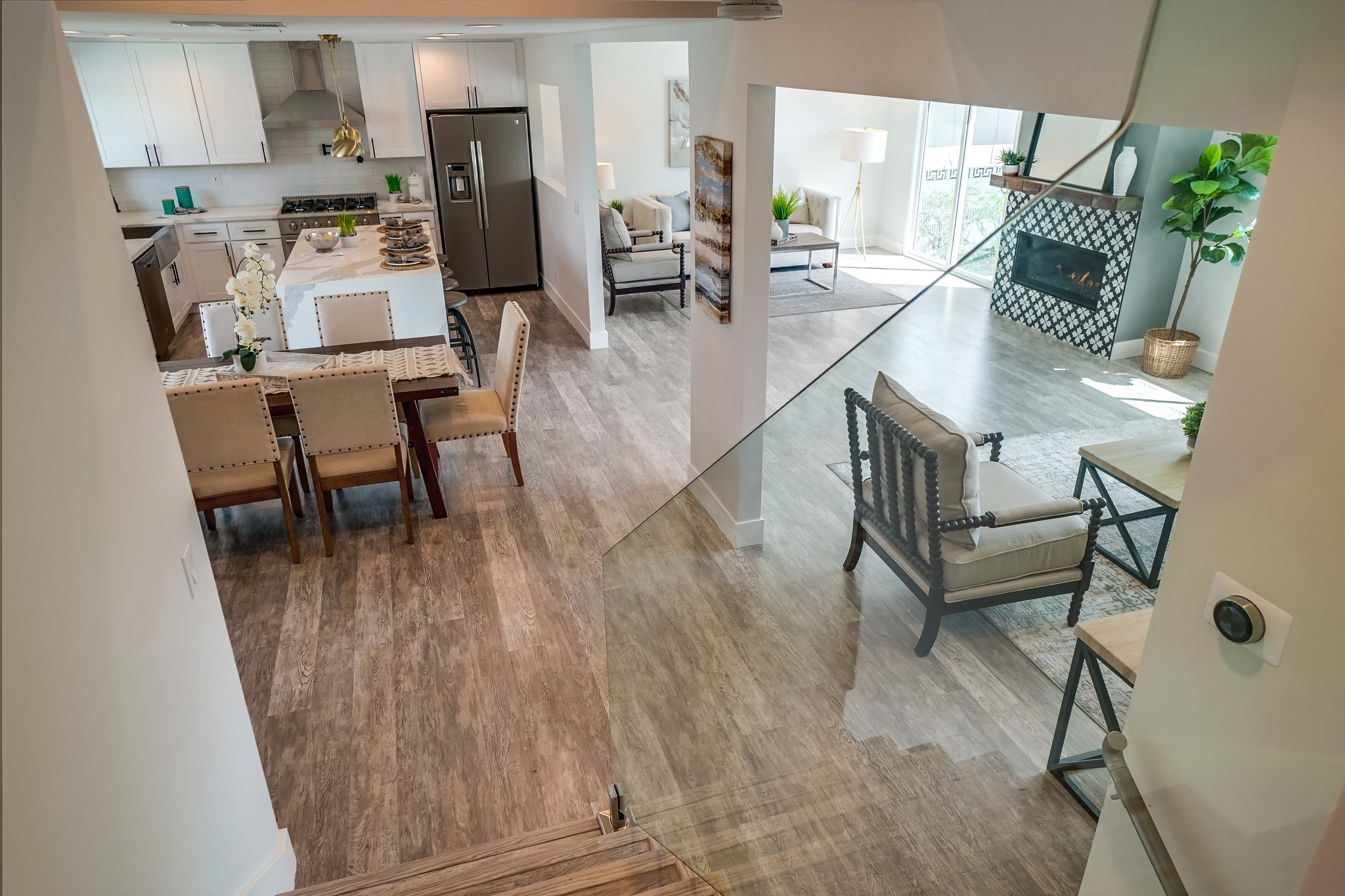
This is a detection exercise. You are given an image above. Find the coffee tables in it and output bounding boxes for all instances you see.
[770,232,839,298]
[1045,430,1192,823]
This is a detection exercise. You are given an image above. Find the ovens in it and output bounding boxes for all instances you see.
[281,234,299,261]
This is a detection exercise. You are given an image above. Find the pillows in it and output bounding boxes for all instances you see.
[871,371,978,550]
[599,203,634,262]
[656,190,690,232]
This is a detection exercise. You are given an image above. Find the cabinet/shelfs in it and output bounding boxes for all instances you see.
[354,43,519,161]
[68,42,286,333]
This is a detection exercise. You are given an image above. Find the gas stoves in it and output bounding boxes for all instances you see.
[277,192,380,236]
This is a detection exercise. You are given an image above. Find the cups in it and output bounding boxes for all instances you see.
[175,186,194,209]
[162,199,175,215]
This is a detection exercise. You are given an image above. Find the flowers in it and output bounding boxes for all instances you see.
[223,241,276,373]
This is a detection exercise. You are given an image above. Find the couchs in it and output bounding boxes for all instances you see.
[633,184,840,280]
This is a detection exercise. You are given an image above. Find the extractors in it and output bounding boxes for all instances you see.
[262,40,365,128]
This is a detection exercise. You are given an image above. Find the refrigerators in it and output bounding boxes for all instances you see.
[430,112,539,294]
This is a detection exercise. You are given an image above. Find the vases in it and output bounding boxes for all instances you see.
[1112,147,1138,196]
[232,348,268,374]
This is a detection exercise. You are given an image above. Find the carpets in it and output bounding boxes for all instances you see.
[826,418,1185,734]
[656,268,908,321]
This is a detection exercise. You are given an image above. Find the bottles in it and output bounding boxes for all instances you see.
[771,222,783,240]
[407,172,426,201]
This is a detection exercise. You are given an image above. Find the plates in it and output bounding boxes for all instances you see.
[387,235,420,239]
[386,260,421,266]
[385,224,418,229]
[386,244,425,252]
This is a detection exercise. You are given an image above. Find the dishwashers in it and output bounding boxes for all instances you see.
[132,246,176,356]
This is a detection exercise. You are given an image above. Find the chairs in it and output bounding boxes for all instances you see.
[600,220,685,316]
[166,291,530,564]
[845,387,1106,657]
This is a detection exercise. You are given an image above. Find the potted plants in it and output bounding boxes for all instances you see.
[384,173,404,202]
[337,213,359,248]
[772,188,811,238]
[994,148,1039,176]
[1142,133,1278,379]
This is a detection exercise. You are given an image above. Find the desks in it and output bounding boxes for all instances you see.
[157,334,474,518]
[275,222,450,346]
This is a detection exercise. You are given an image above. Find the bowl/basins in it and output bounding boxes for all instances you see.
[304,232,340,254]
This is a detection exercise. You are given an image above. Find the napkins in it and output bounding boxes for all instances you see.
[384,218,430,264]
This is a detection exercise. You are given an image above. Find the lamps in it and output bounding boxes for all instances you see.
[840,127,888,260]
[597,162,616,202]
[318,35,366,158]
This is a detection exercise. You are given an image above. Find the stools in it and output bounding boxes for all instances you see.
[436,254,482,387]
